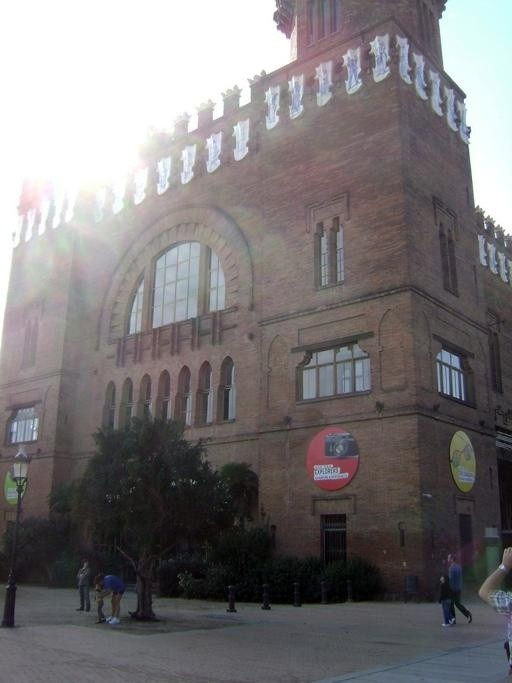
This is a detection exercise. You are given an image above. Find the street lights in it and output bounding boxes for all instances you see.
[0,445,30,629]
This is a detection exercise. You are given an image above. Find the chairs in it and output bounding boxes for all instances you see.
[402,576,427,605]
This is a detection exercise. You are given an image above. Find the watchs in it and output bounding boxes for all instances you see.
[498,564,510,575]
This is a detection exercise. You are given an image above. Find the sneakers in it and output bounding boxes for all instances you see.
[105,616,121,624]
[440,612,472,628]
[76,607,90,612]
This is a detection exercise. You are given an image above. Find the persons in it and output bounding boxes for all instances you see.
[446,553,472,624]
[74,556,92,612]
[477,545,512,676]
[94,588,106,624]
[94,573,126,625]
[437,572,455,627]
